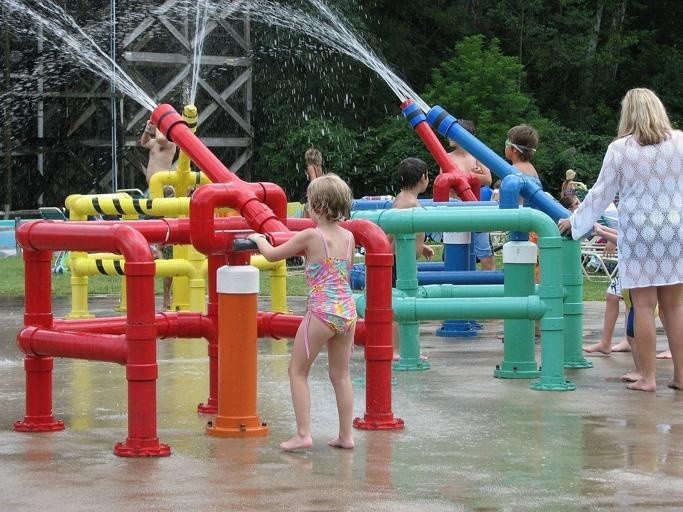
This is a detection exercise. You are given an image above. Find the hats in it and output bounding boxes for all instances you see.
[566,169,576,180]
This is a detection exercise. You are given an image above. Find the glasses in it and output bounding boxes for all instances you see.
[505,139,523,154]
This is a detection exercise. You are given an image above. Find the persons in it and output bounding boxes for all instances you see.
[495,122,541,340]
[556,88,683,392]
[246,173,359,451]
[304,149,325,218]
[140,118,176,184]
[439,135,495,270]
[582,221,673,381]
[160,244,176,312]
[561,167,577,196]
[561,193,606,243]
[384,158,435,287]
[490,178,501,202]
